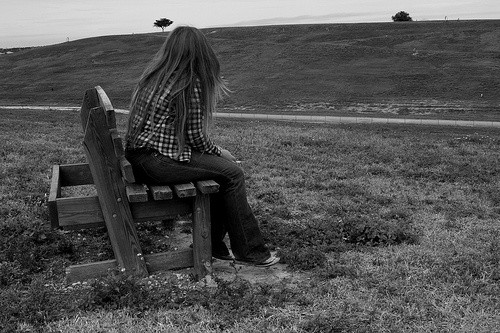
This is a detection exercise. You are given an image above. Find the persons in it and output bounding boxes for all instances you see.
[125,25,280,266]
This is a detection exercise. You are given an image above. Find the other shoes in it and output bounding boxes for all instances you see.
[212,254,234,263]
[237,257,280,266]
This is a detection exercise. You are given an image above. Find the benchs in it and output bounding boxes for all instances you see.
[46,83,224,285]
